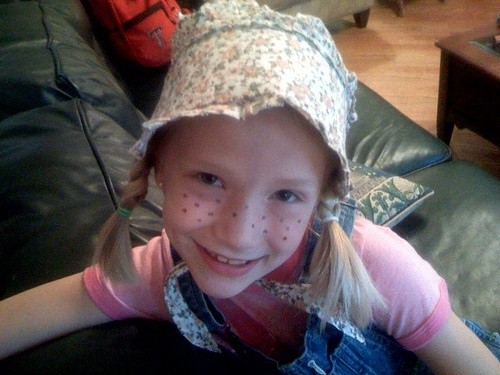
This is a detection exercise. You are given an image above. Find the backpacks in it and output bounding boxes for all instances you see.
[77,0,193,99]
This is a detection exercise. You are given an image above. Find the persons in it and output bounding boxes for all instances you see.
[0,0,500,375]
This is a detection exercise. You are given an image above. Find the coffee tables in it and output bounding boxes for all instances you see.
[432,23,500,155]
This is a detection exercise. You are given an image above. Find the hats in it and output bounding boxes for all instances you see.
[127,1,358,200]
